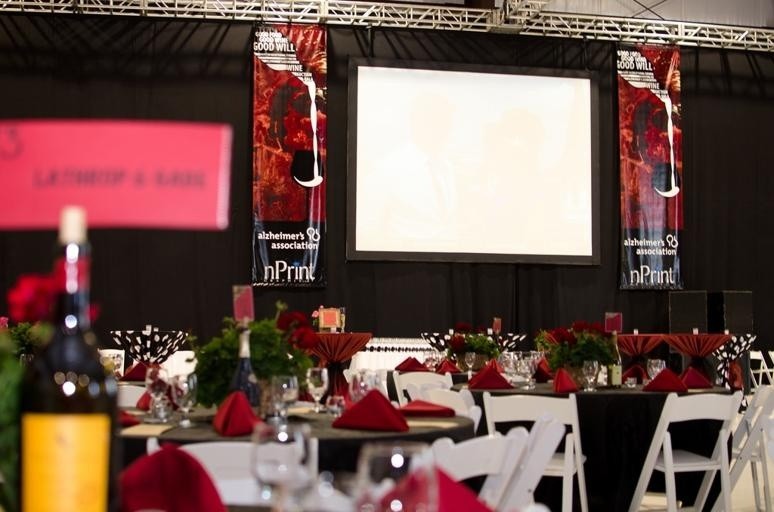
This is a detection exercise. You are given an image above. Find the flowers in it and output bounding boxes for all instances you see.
[1,257,99,511]
[446,321,502,361]
[535,320,620,370]
[200,301,325,389]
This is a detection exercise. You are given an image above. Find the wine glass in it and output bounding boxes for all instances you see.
[461,346,665,392]
[137,366,199,429]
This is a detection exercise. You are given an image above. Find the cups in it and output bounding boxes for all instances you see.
[251,418,440,512]
[422,349,445,371]
[271,366,386,419]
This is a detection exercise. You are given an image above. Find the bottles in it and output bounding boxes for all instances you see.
[24,199,118,510]
[607,330,624,388]
[232,326,262,421]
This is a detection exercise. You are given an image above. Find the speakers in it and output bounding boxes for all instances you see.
[656,290,708,368]
[708,289,753,334]
